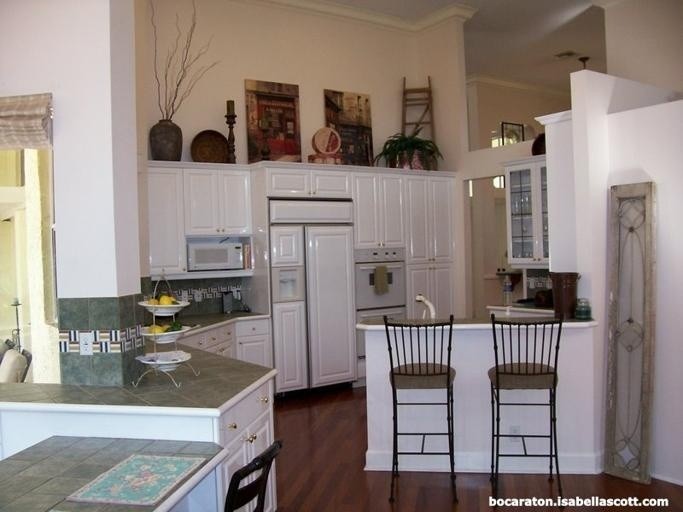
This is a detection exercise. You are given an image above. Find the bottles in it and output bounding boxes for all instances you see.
[573,297,593,323]
[500,275,514,307]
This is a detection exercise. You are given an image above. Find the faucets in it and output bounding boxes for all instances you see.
[415,294,436,320]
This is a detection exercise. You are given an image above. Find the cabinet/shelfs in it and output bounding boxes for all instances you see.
[214,377,276,512]
[401,168,456,266]
[405,262,456,320]
[250,160,351,202]
[346,165,404,248]
[178,332,209,351]
[184,161,249,236]
[144,158,187,279]
[500,154,550,271]
[206,321,237,361]
[233,317,271,370]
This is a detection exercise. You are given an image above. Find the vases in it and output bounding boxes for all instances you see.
[145,116,185,164]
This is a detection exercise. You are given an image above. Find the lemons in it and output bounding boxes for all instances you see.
[149,325,171,332]
[148,296,176,305]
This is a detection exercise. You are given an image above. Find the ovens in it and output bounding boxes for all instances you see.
[353,246,410,358]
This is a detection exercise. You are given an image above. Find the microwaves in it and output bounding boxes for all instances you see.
[186,240,245,272]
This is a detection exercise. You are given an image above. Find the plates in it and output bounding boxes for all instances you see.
[134,351,192,373]
[137,300,191,319]
[141,324,190,345]
[190,129,231,164]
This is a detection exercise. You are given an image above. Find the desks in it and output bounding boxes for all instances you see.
[355,316,600,475]
[0,432,229,511]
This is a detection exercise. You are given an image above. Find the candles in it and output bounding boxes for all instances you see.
[224,99,234,114]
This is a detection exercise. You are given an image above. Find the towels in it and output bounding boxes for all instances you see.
[372,264,390,297]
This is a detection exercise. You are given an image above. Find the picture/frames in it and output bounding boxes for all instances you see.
[500,121,524,147]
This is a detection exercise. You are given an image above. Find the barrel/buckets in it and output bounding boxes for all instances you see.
[549,271,579,320]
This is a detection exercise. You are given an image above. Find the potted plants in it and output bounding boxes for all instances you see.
[372,129,444,173]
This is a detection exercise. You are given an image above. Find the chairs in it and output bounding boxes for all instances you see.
[381,311,459,505]
[223,437,284,512]
[485,311,566,511]
